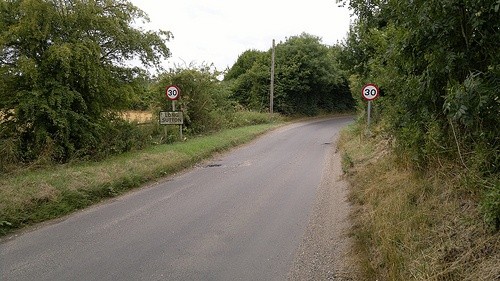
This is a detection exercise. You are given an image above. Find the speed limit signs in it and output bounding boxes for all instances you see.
[362,84,379,101]
[166,86,178,100]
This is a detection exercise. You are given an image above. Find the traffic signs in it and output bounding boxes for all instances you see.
[159,111,184,125]
